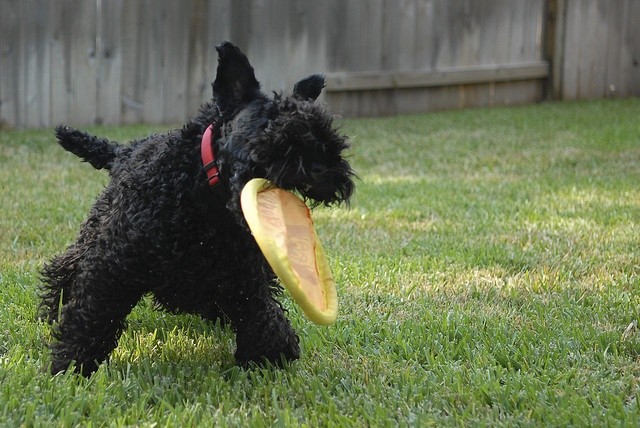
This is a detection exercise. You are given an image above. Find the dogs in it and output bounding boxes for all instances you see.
[34,38,363,375]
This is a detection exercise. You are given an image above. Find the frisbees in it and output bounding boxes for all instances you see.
[240,178,338,324]
